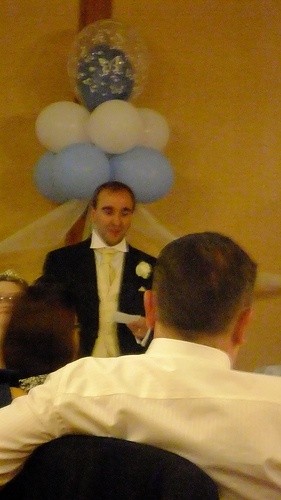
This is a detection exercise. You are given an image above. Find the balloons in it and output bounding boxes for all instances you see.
[33,19,174,204]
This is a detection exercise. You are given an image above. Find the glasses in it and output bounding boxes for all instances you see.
[0,296,14,305]
[75,323,81,332]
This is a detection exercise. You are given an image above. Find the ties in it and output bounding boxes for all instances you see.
[96,248,117,358]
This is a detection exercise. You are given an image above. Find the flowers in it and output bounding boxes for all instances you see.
[135,261,151,279]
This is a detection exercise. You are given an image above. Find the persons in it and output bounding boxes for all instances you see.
[0,181,281,385]
[0,231,281,500]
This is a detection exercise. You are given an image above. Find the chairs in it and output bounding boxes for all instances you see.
[0,434,219,500]
